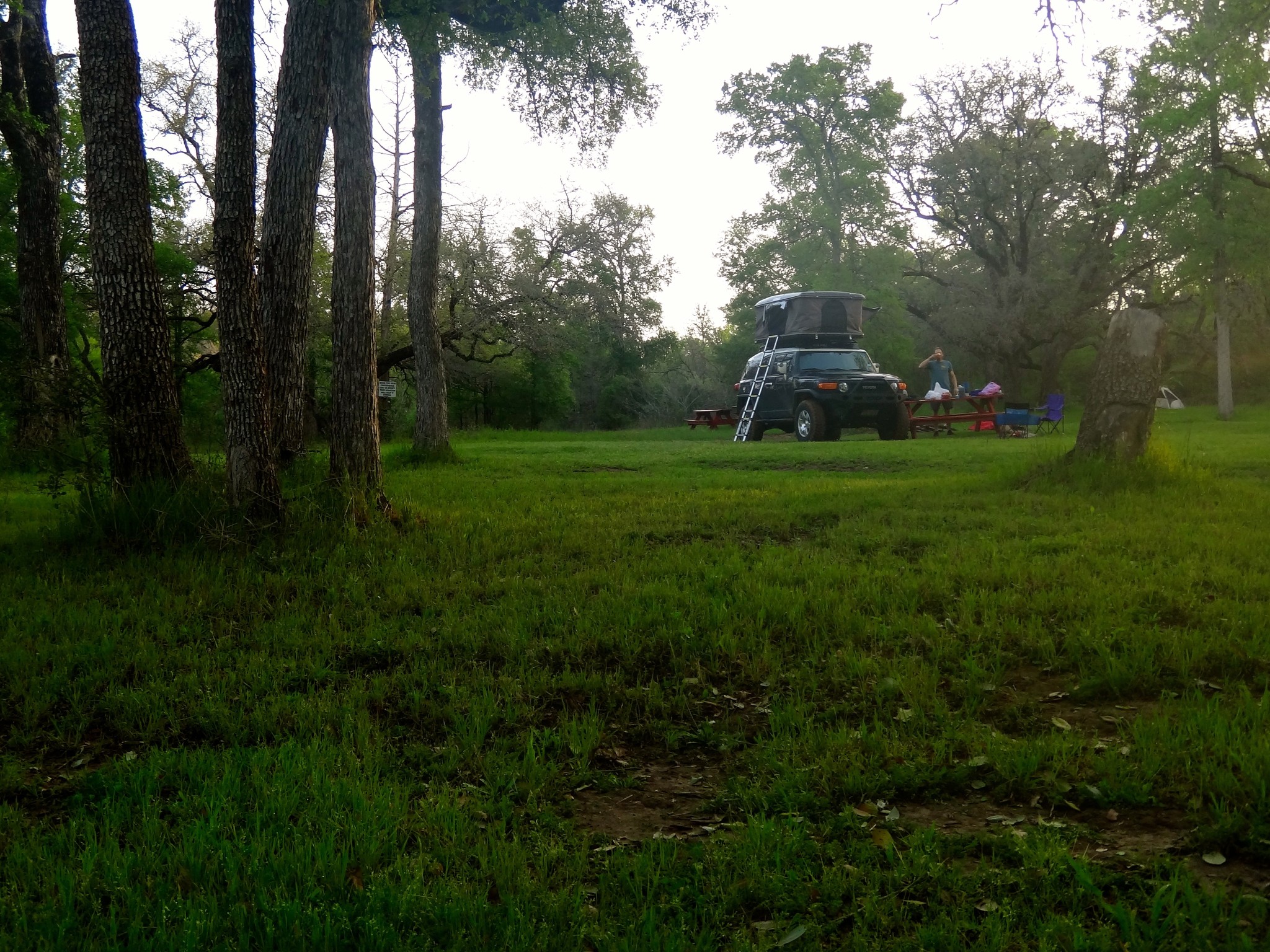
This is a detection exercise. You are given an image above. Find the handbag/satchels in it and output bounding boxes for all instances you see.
[925,382,950,400]
[979,382,1001,395]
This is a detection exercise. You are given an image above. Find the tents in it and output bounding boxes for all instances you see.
[753,291,866,344]
[1155,387,1185,409]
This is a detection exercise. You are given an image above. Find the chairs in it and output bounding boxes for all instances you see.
[1033,394,1065,438]
[1000,403,1030,440]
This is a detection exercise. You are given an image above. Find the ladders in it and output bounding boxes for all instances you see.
[734,335,779,440]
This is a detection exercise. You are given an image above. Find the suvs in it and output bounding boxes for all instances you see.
[734,346,910,441]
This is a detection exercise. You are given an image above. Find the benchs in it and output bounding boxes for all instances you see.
[913,410,990,422]
[909,412,1006,423]
[683,420,711,422]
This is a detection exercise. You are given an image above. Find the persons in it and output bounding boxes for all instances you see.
[918,346,958,438]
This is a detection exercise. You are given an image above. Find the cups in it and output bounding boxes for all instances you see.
[935,353,941,359]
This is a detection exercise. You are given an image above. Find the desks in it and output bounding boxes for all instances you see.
[691,409,736,431]
[901,392,1004,439]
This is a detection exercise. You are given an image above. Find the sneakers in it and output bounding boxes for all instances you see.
[933,431,939,437]
[947,430,957,436]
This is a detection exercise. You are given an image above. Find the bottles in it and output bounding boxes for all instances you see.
[957,385,963,397]
